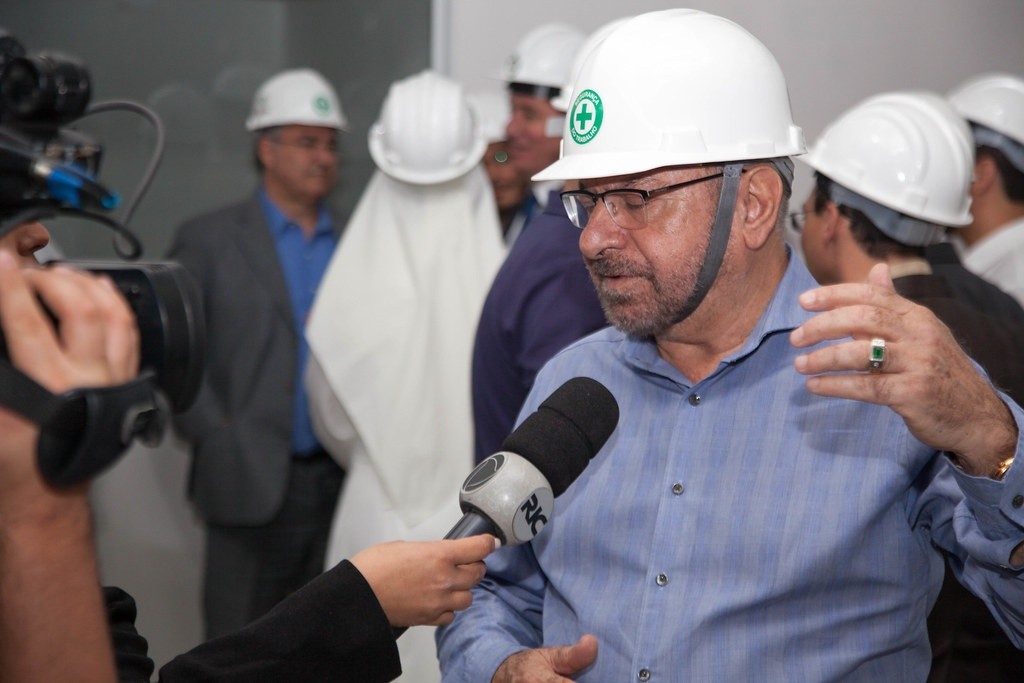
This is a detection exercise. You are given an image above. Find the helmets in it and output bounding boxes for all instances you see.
[366,70,488,187]
[952,77,1023,142]
[528,10,806,180]
[503,25,589,88]
[796,93,973,227]
[245,68,350,132]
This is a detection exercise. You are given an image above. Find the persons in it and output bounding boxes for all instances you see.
[0,7,1024,683]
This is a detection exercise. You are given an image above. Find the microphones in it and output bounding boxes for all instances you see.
[443,376,620,546]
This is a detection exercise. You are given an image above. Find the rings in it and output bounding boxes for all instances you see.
[870,337,885,375]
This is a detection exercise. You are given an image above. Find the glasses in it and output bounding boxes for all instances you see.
[560,168,746,230]
[791,208,825,231]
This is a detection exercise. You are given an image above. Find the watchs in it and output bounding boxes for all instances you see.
[989,457,1015,481]
